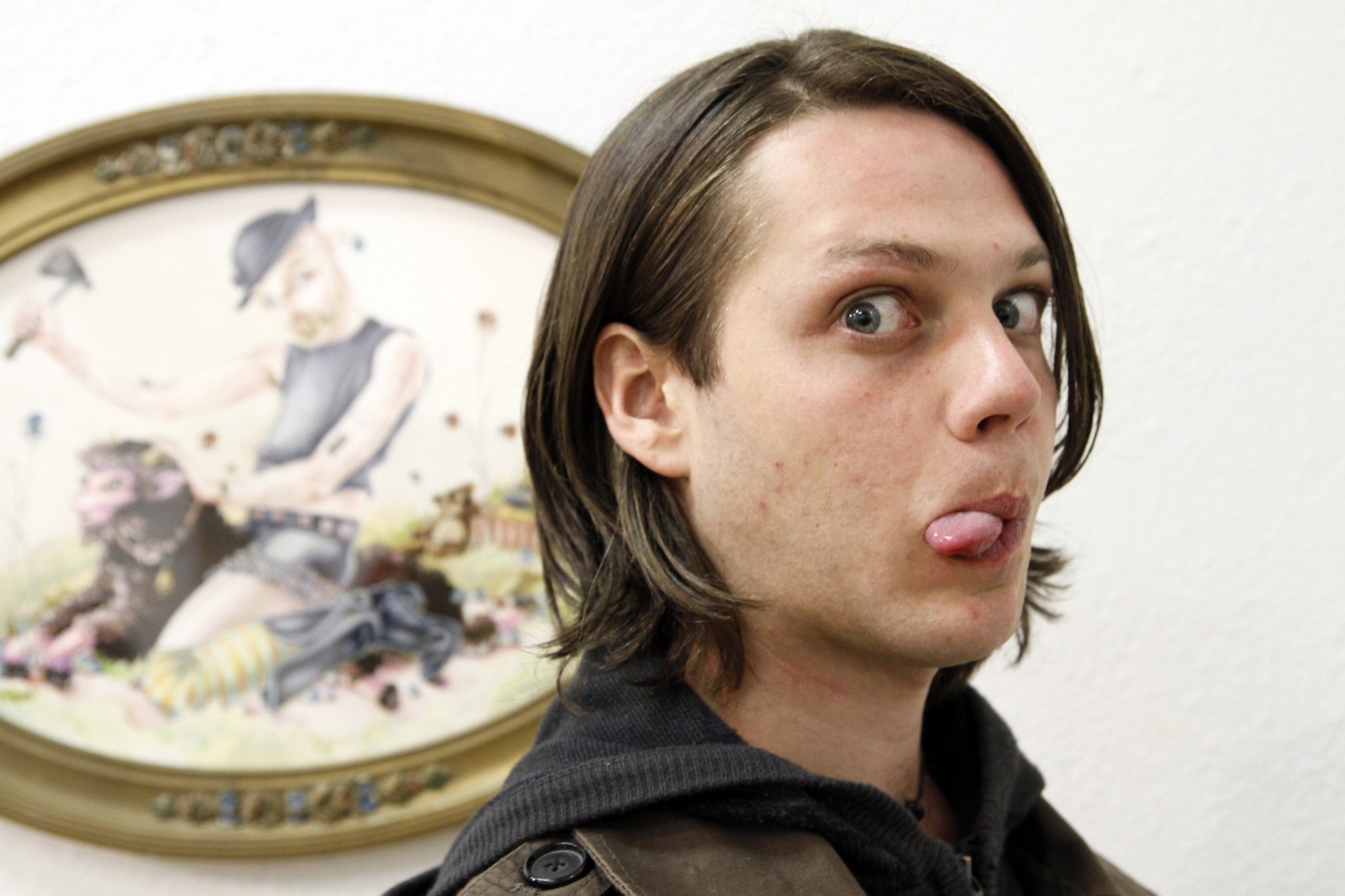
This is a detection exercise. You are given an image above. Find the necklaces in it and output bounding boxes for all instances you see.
[904,769,926,822]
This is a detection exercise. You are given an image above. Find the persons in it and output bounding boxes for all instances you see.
[383,27,1154,896]
